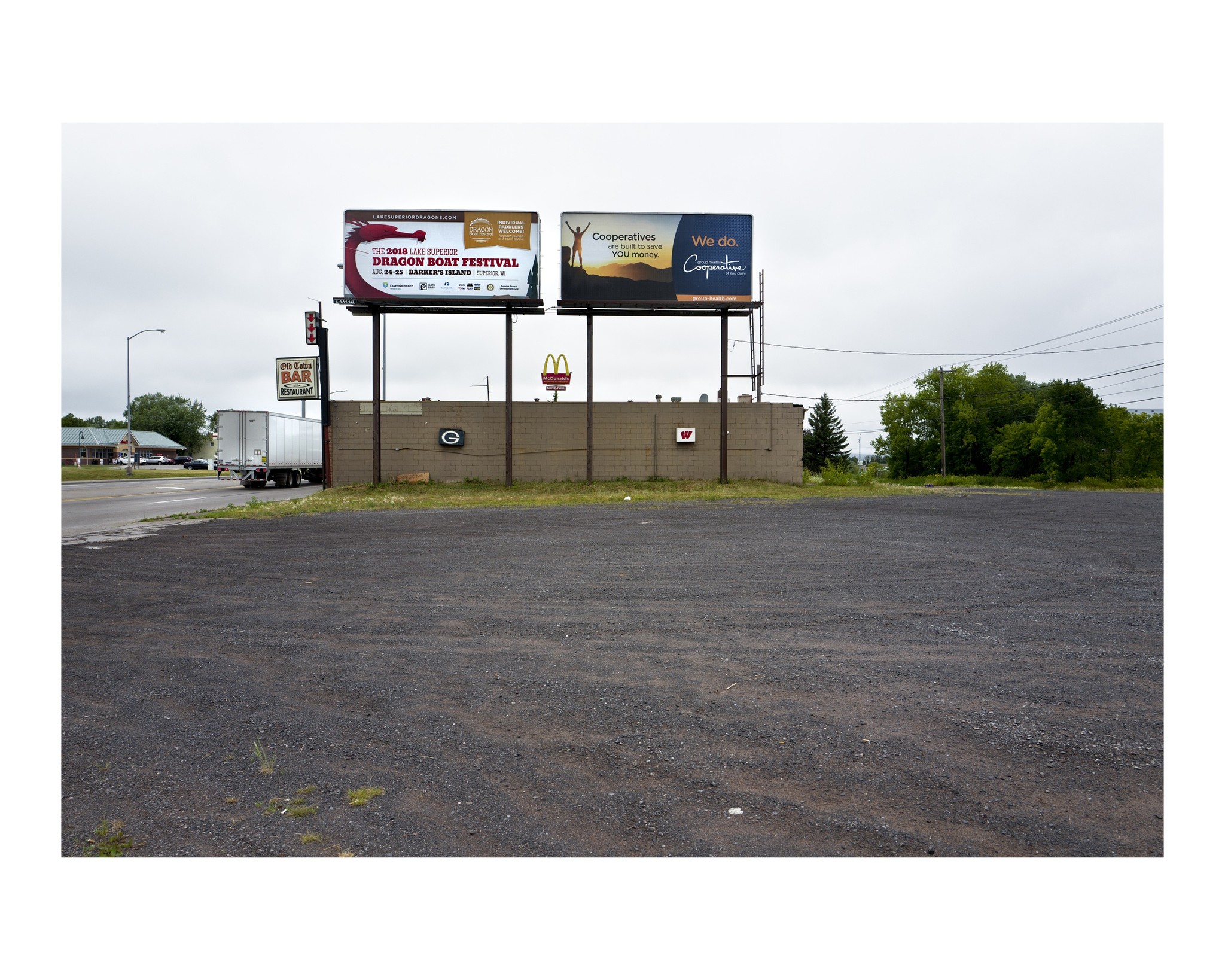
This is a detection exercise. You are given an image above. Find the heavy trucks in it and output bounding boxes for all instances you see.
[215,410,322,489]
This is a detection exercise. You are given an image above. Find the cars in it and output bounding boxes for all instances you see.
[117,455,147,466]
[146,455,169,465]
[162,456,176,465]
[171,456,193,465]
[183,458,229,471]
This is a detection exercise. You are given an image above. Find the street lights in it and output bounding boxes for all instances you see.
[126,329,166,475]
[78,431,84,469]
